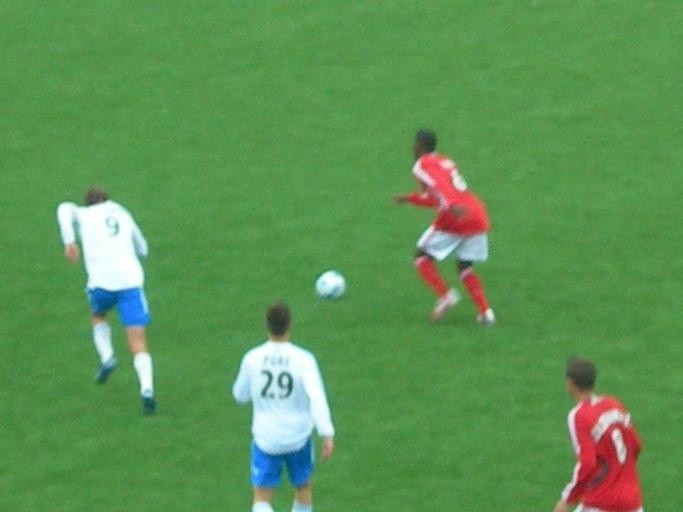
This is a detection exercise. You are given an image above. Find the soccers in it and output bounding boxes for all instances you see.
[315,269,346,300]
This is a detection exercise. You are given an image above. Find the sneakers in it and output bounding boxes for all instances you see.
[429,288,462,321]
[141,388,156,414]
[476,307,495,326]
[94,354,120,385]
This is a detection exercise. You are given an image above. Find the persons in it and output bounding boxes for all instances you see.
[552,355,645,512]
[56,188,157,415]
[389,126,496,324]
[231,299,335,512]
